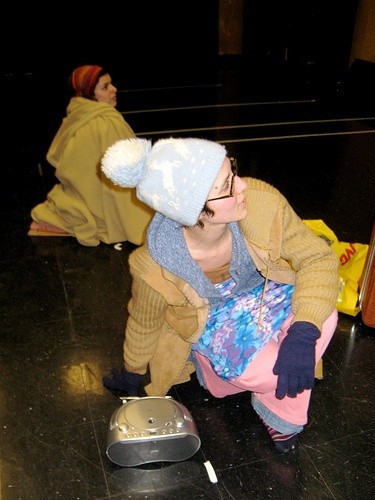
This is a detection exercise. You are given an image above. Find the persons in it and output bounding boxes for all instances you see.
[98,137,340,456]
[30,66,156,247]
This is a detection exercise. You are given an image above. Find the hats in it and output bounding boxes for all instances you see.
[101,138,228,227]
[71,65,104,99]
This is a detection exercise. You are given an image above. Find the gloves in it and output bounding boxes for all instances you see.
[272,321,322,400]
[102,367,150,396]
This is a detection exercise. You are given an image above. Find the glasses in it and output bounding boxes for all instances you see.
[206,156,238,203]
